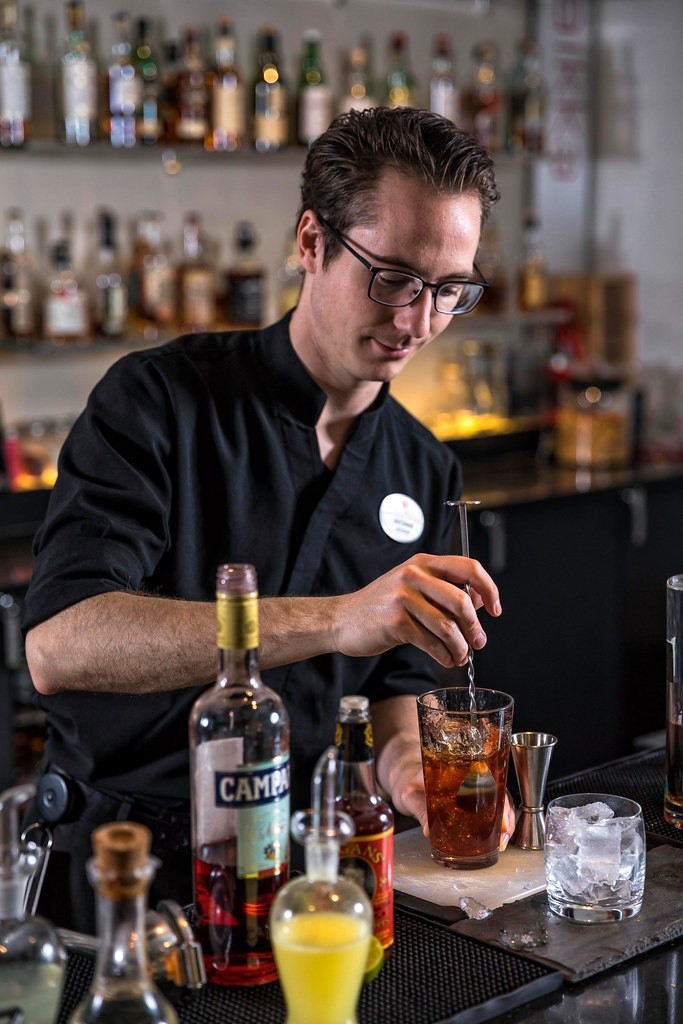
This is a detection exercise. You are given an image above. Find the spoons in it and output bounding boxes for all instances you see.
[444,500,495,813]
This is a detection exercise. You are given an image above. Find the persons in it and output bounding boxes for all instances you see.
[20,105,515,938]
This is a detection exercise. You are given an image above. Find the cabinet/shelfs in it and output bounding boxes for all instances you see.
[462,476,682,782]
[0,121,571,545]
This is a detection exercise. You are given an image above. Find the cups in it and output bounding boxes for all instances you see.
[664,574,683,829]
[544,792,646,923]
[509,732,558,850]
[416,687,514,871]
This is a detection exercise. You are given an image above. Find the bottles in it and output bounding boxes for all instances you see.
[190,565,290,986]
[0,204,551,349]
[0,785,68,1024]
[70,821,179,1024]
[269,812,374,1024]
[317,697,394,960]
[0,0,553,154]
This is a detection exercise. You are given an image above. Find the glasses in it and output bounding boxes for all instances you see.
[314,211,492,315]
